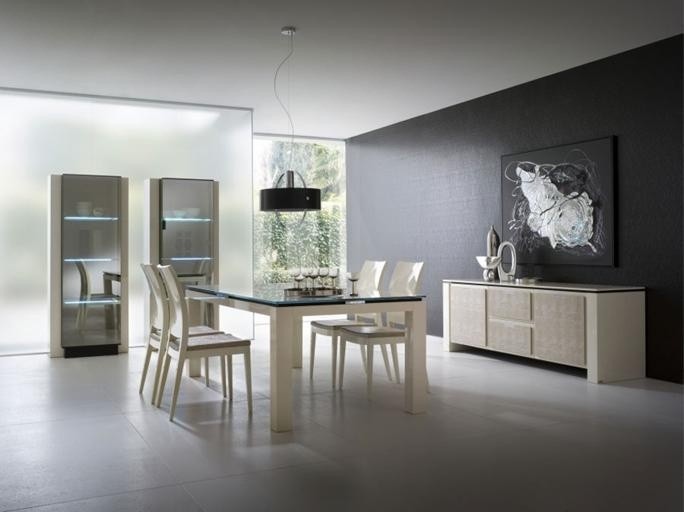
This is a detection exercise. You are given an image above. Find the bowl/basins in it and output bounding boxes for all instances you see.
[474,256,503,269]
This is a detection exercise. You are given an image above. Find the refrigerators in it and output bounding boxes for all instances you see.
[57,174,122,358]
[150,177,214,332]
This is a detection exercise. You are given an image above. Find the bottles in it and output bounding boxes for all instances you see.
[486,224,499,258]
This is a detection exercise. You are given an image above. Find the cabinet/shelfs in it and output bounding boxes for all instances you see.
[442,280,646,384]
[49,173,128,358]
[142,177,219,352]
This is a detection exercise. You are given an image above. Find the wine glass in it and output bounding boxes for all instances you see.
[345,271,360,297]
[328,267,339,290]
[289,267,318,295]
[318,267,328,290]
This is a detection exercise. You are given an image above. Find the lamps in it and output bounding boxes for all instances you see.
[260,27,320,231]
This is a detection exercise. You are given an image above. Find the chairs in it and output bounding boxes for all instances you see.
[74,259,206,335]
[155,265,253,422]
[308,262,392,387]
[337,261,430,399]
[139,264,226,404]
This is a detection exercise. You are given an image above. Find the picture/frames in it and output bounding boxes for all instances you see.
[501,134,619,268]
